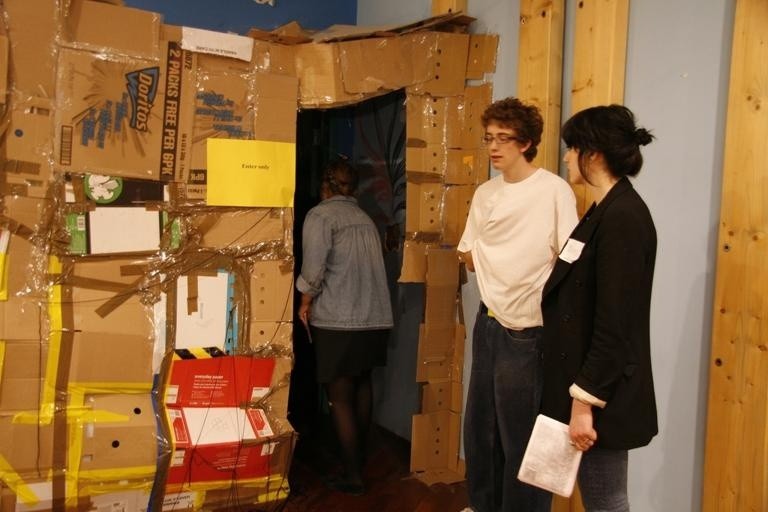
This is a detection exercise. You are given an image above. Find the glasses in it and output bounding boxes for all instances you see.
[482,134,521,145]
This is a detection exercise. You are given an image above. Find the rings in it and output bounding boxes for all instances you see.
[569,440,574,444]
[584,438,589,443]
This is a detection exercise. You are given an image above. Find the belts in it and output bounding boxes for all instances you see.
[479,301,494,317]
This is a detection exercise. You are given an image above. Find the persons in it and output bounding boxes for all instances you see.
[453,95,580,511]
[293,160,396,497]
[537,103,659,511]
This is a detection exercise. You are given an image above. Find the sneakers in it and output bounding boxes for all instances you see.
[324,477,364,492]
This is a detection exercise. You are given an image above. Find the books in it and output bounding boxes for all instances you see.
[516,414,583,499]
